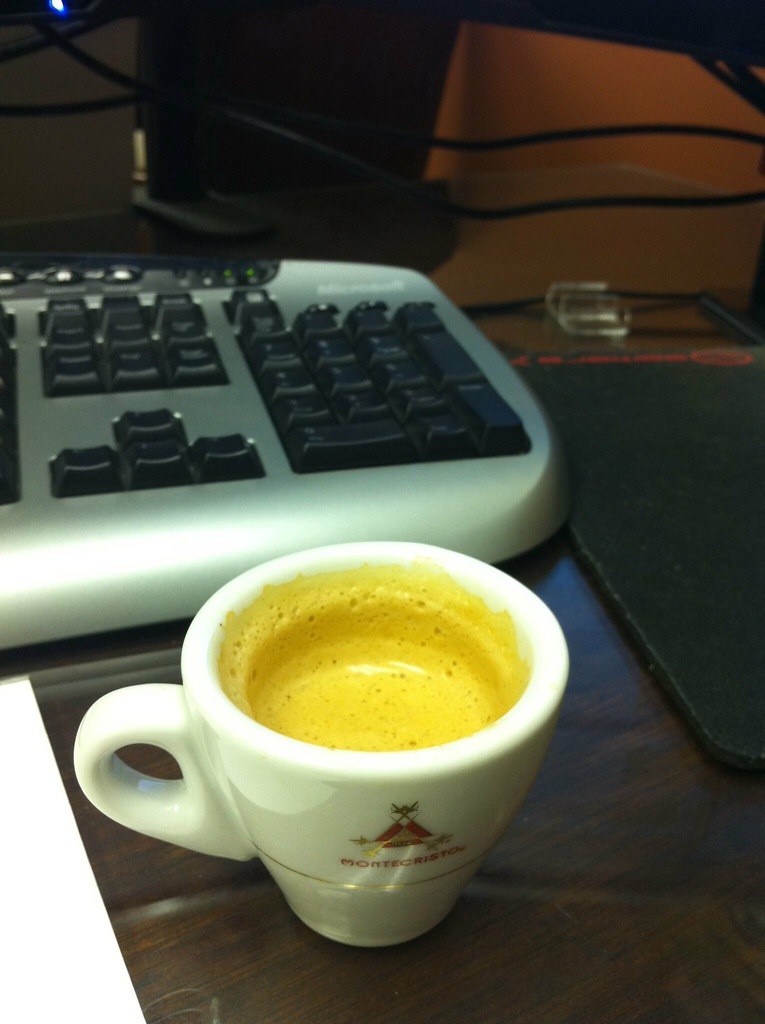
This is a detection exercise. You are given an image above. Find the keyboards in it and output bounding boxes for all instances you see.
[0,256,568,653]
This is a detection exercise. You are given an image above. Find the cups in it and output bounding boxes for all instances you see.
[73,541,569,947]
